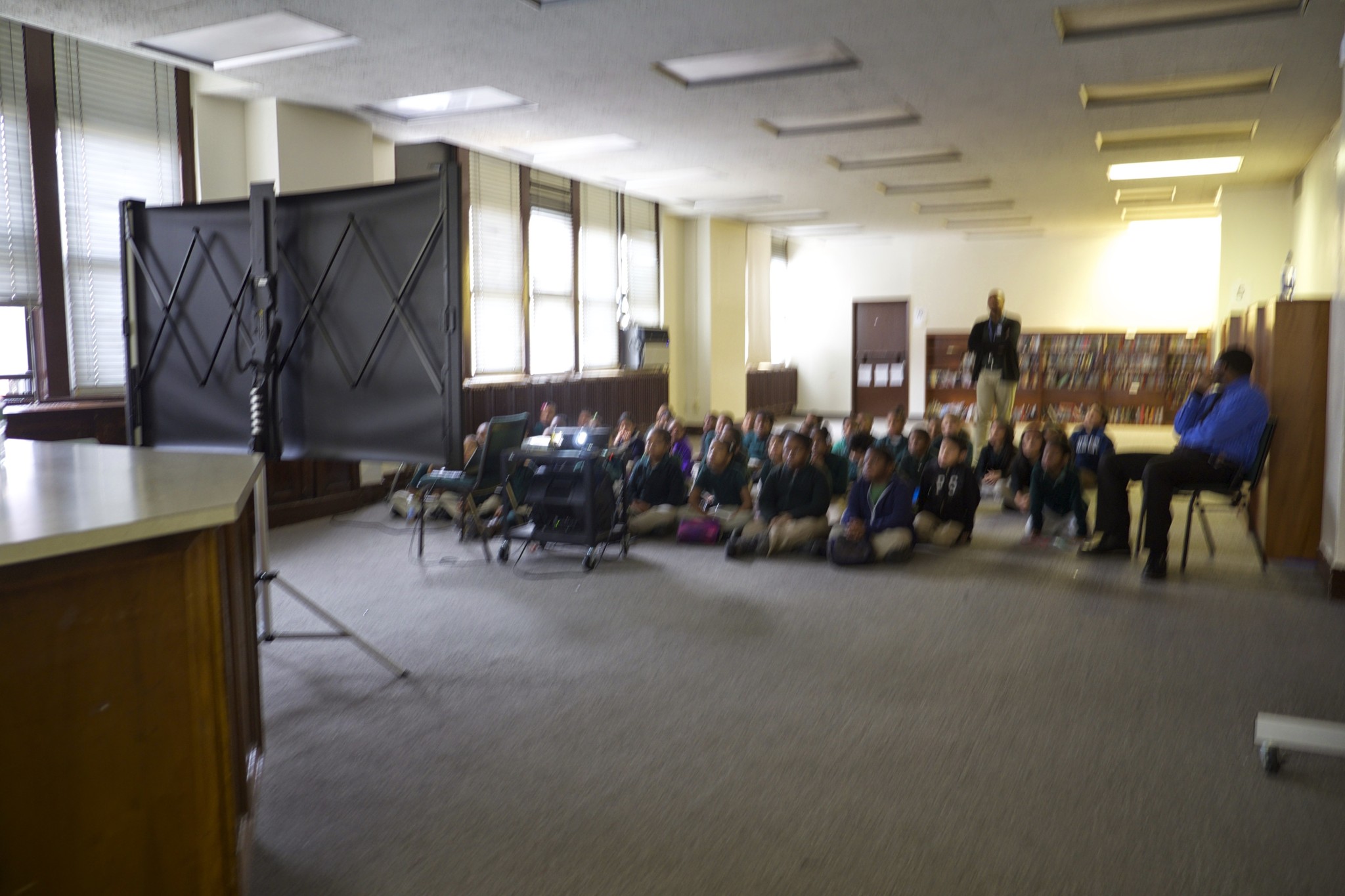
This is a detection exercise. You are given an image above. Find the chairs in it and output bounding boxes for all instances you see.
[415,411,532,564]
[1134,421,1278,575]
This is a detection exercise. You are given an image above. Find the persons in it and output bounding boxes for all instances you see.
[968,291,1023,469]
[1079,342,1270,581]
[391,398,1115,564]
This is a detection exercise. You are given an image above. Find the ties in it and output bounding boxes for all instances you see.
[1197,388,1224,426]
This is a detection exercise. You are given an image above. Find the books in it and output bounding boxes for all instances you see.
[928,335,1207,425]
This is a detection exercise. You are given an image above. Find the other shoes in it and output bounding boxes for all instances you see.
[1016,534,1055,552]
[1000,497,1017,514]
[806,532,829,557]
[725,526,742,556]
[1076,532,1131,559]
[1141,550,1168,580]
[826,534,877,566]
[675,516,725,547]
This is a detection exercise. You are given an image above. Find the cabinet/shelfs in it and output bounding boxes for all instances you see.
[924,300,1330,563]
